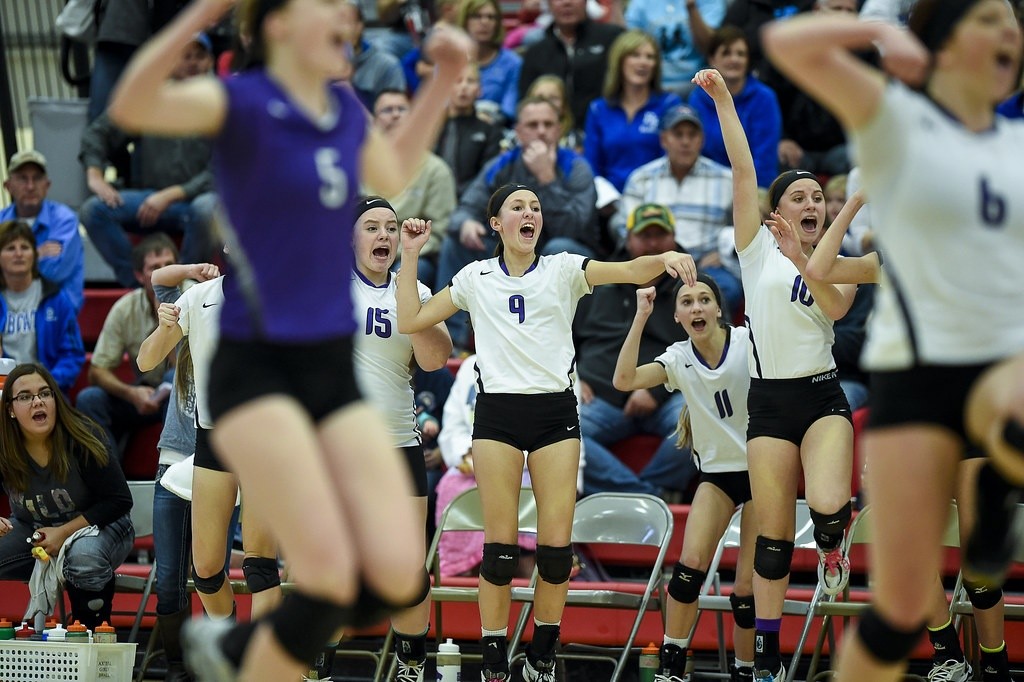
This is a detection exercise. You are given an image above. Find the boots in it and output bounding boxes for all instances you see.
[204,603,236,627]
[154,597,189,682]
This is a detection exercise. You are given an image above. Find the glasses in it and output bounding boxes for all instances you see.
[12,391,55,405]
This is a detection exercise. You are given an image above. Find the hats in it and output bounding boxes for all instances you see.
[9,149,46,173]
[626,202,675,234]
[659,104,703,132]
[193,32,209,48]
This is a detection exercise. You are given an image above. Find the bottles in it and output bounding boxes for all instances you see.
[685,650,694,682]
[638,642,660,682]
[0,617,117,682]
[435,638,461,682]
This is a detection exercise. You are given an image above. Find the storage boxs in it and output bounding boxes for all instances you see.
[0,640,139,682]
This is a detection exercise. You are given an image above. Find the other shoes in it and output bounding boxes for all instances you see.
[731,665,754,682]
[180,619,239,682]
[653,671,691,682]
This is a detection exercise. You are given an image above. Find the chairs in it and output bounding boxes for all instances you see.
[952,504,1024,682]
[96,482,156,682]
[509,492,673,682]
[805,499,962,682]
[386,486,539,682]
[686,499,844,682]
[136,487,251,682]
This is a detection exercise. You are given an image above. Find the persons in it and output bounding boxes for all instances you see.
[0,221,87,402]
[691,69,857,682]
[0,150,85,320]
[611,274,774,682]
[78,202,701,682]
[0,492,0,495]
[346,193,453,592]
[396,182,700,682]
[0,362,139,643]
[56,0,881,356]
[758,0,1024,682]
[108,0,474,682]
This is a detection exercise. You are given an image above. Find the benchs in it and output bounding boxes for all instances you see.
[0,289,1024,660]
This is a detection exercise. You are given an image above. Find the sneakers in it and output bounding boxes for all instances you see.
[754,662,786,682]
[928,655,974,682]
[816,530,850,596]
[478,667,512,682]
[392,650,427,682]
[522,656,556,682]
